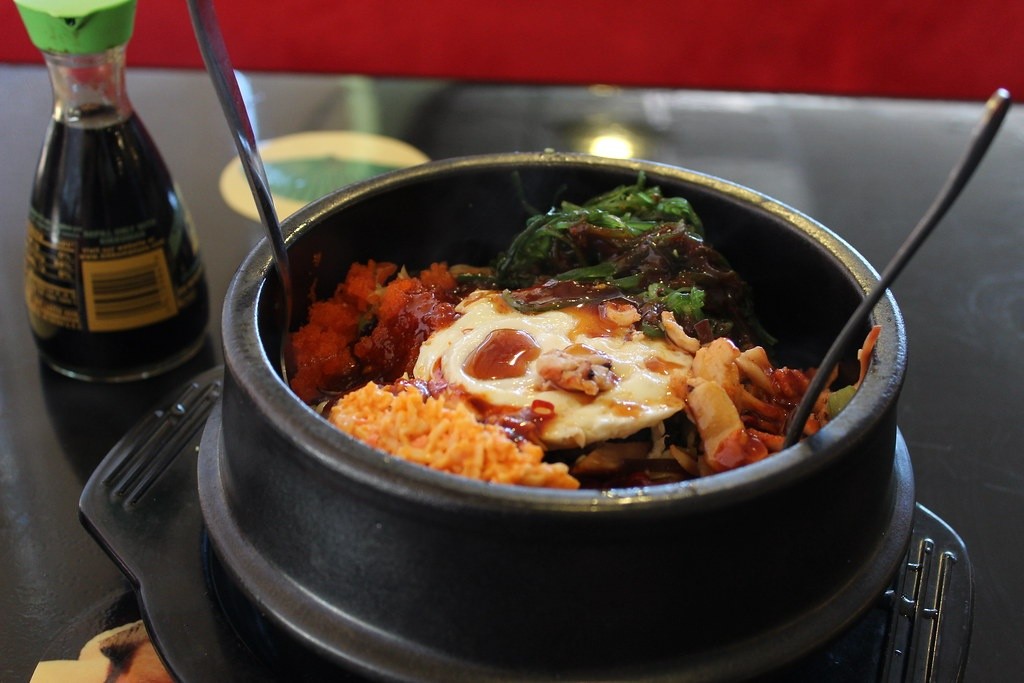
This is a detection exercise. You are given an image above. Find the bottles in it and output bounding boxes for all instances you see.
[17,0,211,383]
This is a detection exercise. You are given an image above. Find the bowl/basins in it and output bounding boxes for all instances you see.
[201,147,916,683]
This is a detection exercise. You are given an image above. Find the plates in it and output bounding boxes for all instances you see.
[71,366,972,683]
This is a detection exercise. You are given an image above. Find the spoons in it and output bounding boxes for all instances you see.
[186,0,298,389]
